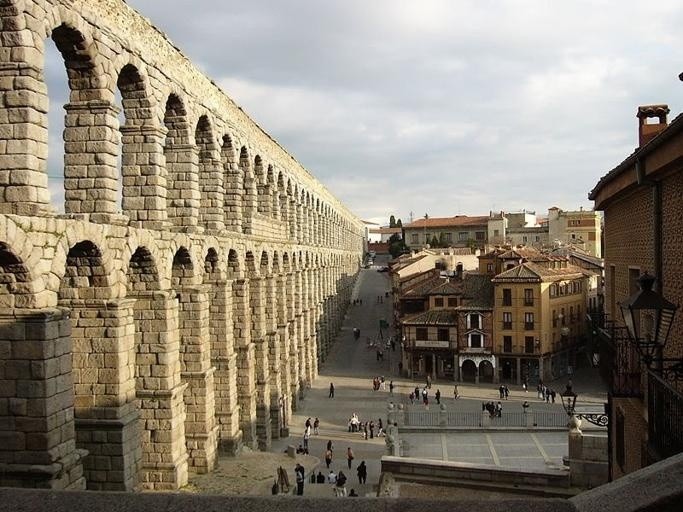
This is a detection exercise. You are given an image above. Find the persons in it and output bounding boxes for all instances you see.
[453,385,458,400]
[293,464,304,495]
[525,377,528,393]
[535,383,555,403]
[302,429,309,455]
[421,384,428,405]
[327,470,337,484]
[426,373,431,389]
[316,471,324,483]
[356,460,366,484]
[345,447,353,469]
[434,389,440,405]
[328,382,334,398]
[481,400,502,418]
[296,444,303,455]
[325,449,332,469]
[314,418,320,435]
[389,380,393,397]
[413,385,419,400]
[498,386,504,399]
[347,413,387,440]
[311,471,314,483]
[371,375,386,391]
[327,439,332,450]
[398,362,402,373]
[333,470,347,498]
[409,392,415,404]
[349,488,358,497]
[304,418,312,434]
[504,386,508,400]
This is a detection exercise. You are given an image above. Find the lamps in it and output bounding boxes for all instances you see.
[560,383,610,427]
[616,270,683,382]
